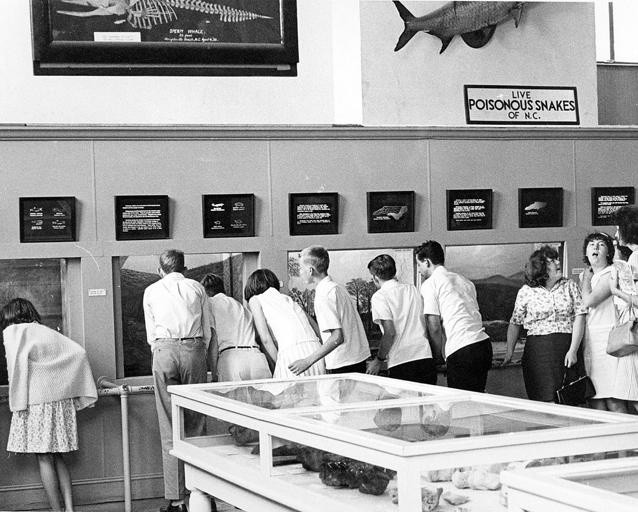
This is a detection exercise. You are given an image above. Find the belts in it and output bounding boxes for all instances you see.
[219,345,259,353]
[155,337,203,341]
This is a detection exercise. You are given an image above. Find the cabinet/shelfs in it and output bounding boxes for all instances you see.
[167,372,638,512]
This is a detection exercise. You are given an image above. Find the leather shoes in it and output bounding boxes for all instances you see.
[160,502,187,512]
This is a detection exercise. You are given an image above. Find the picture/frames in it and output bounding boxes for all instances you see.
[463,84,580,126]
[31,1,299,65]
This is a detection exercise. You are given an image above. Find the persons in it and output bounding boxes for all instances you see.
[197,272,273,384]
[141,250,214,511]
[0,298,98,510]
[578,231,620,413]
[414,239,493,393]
[608,205,638,414]
[243,268,327,380]
[199,379,457,439]
[285,245,373,376]
[366,252,437,384]
[488,243,588,408]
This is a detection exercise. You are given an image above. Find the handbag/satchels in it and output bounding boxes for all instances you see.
[605,318,638,357]
[556,366,596,405]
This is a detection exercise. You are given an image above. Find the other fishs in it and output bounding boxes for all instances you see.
[392,0,525,55]
